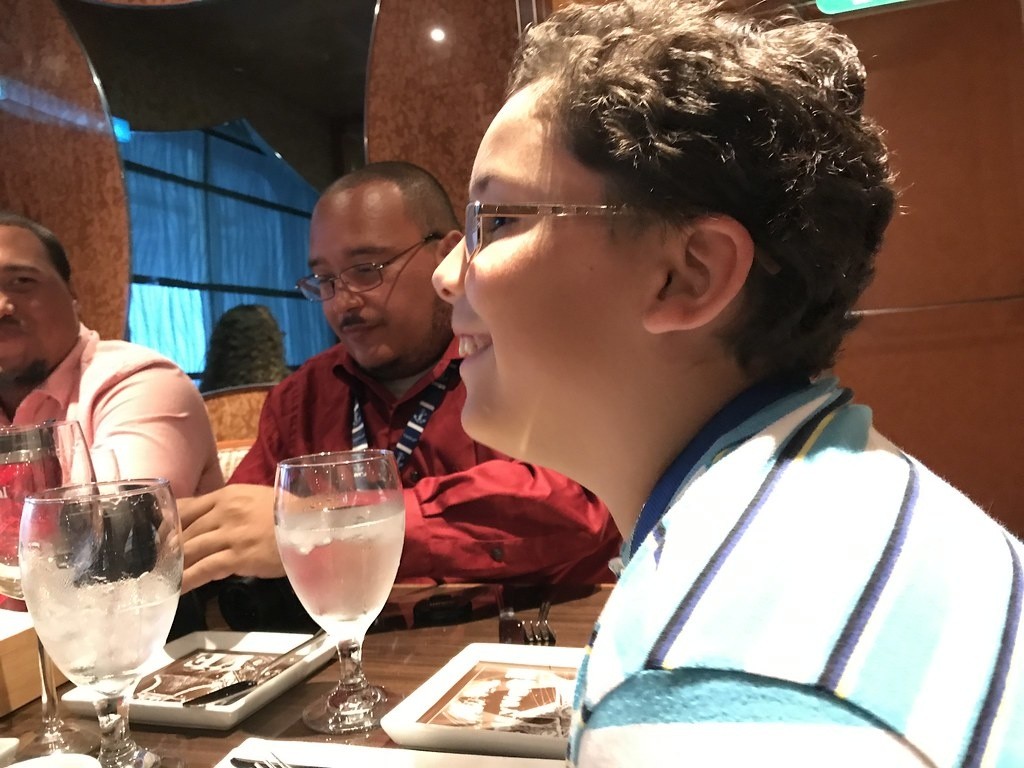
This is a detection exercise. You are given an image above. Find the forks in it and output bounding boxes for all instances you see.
[518,585,557,646]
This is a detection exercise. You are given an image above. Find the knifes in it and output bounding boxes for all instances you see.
[183,628,329,708]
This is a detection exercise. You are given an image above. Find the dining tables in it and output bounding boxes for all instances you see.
[0,581,618,768]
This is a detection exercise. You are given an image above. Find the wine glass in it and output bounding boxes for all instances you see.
[19,479,184,768]
[0,420,106,763]
[273,449,406,735]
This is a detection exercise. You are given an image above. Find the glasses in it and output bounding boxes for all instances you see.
[462,201,656,253]
[296,232,445,302]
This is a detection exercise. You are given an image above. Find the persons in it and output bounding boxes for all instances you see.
[430,0,1024,768]
[152,162,623,596]
[1,209,227,499]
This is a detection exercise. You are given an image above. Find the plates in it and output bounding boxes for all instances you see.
[379,640,590,760]
[211,737,571,766]
[59,630,338,731]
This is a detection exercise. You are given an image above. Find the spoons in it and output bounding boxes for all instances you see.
[514,698,577,730]
